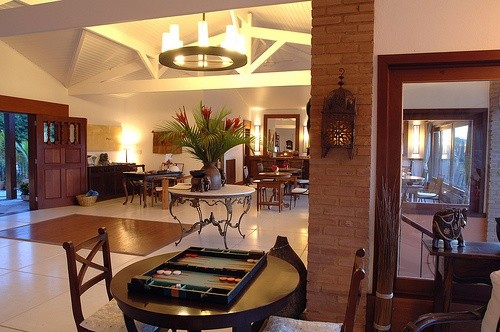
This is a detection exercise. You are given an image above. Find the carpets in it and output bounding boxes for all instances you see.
[0,213,207,257]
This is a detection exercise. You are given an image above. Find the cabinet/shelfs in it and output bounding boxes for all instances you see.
[87,163,138,203]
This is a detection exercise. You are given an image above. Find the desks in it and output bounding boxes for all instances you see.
[402,184,424,203]
[167,183,256,250]
[109,251,301,332]
[420,239,500,332]
[276,167,302,201]
[402,175,425,186]
[258,171,292,210]
[123,170,179,208]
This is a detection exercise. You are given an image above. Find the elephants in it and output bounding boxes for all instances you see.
[432,207,468,250]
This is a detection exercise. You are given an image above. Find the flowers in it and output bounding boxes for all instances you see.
[282,161,289,167]
[272,164,277,171]
[152,98,276,162]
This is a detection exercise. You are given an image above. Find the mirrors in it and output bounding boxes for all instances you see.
[262,113,300,157]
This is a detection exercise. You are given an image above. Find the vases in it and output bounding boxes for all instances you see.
[199,161,222,190]
[285,165,288,169]
[274,166,279,173]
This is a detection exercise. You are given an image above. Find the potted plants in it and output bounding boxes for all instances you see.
[99,153,110,165]
[19,182,31,200]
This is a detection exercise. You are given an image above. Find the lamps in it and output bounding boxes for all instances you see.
[159,10,247,71]
[320,66,354,162]
[413,125,420,154]
[303,126,310,153]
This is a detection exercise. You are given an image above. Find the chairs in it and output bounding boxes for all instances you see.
[402,269,500,332]
[259,247,367,332]
[122,161,445,214]
[63,226,169,332]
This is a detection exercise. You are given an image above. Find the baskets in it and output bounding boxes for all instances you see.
[75,194,97,207]
